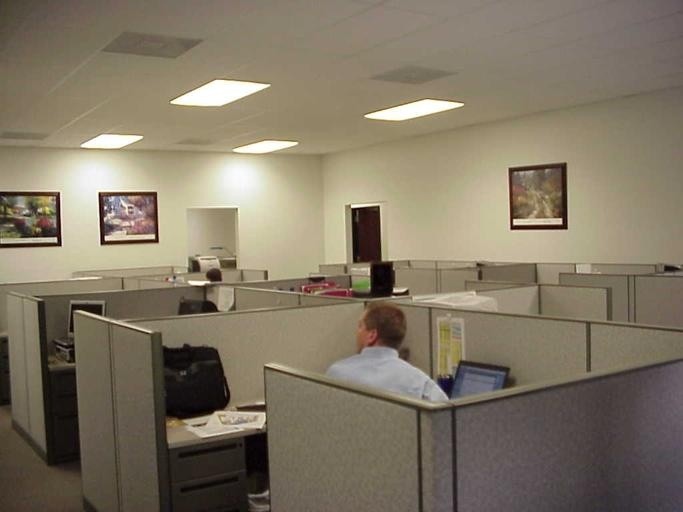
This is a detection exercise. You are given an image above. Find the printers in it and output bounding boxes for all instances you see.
[192,256,220,272]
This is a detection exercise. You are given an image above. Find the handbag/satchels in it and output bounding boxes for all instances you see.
[162,343,231,416]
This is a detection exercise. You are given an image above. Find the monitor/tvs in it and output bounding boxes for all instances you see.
[350,275,371,296]
[448,360,511,400]
[67,300,106,338]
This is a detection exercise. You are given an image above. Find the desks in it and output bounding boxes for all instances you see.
[164,399,266,512]
[0,327,11,405]
[47,353,79,465]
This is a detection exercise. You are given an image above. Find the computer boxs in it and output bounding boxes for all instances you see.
[52,338,75,363]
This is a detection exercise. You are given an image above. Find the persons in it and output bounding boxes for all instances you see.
[206,268,222,281]
[325,303,449,402]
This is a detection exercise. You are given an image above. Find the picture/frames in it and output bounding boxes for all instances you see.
[505,161,570,231]
[0,191,62,248]
[97,190,158,244]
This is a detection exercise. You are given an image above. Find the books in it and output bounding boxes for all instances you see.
[187,409,266,439]
